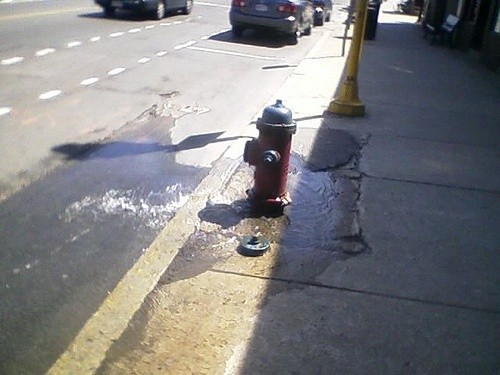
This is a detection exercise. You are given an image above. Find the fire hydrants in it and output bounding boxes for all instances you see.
[243,99,296,217]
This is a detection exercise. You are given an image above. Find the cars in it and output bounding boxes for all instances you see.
[229,0,314,44]
[93,0,194,20]
